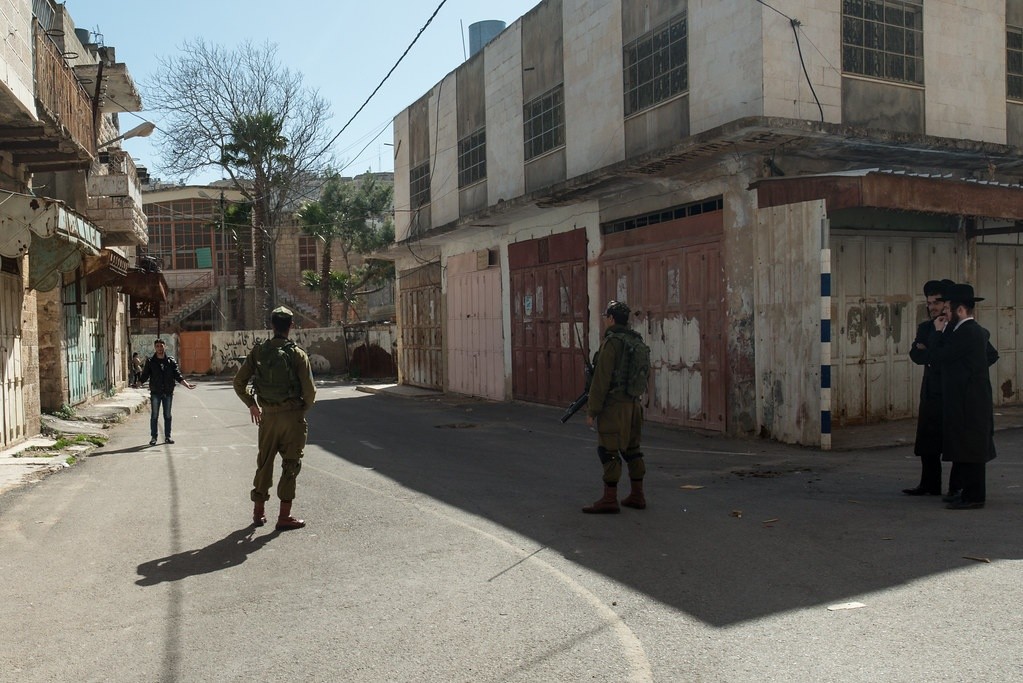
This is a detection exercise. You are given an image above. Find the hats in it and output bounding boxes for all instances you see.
[607,300,632,316]
[936,284,985,301]
[924,279,956,298]
[271,306,293,323]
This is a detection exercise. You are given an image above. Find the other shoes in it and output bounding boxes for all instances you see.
[902,485,941,495]
[150,437,157,445]
[165,436,174,443]
[943,495,984,509]
[945,489,962,502]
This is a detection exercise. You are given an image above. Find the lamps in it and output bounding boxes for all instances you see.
[97,122,156,149]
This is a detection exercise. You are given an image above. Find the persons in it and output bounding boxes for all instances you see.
[132,352,143,387]
[902,279,1000,510]
[131,339,196,446]
[582,299,651,514]
[233,306,317,530]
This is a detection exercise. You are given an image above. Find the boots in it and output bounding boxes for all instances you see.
[582,483,621,514]
[621,479,646,510]
[253,500,267,526]
[276,501,306,530]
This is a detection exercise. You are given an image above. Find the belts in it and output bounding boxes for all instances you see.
[264,405,302,412]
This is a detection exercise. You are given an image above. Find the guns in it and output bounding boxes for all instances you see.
[559,352,599,424]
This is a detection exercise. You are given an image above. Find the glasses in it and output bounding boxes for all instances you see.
[601,312,614,319]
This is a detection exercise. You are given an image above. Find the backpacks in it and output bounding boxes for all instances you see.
[607,331,651,398]
[252,338,298,403]
